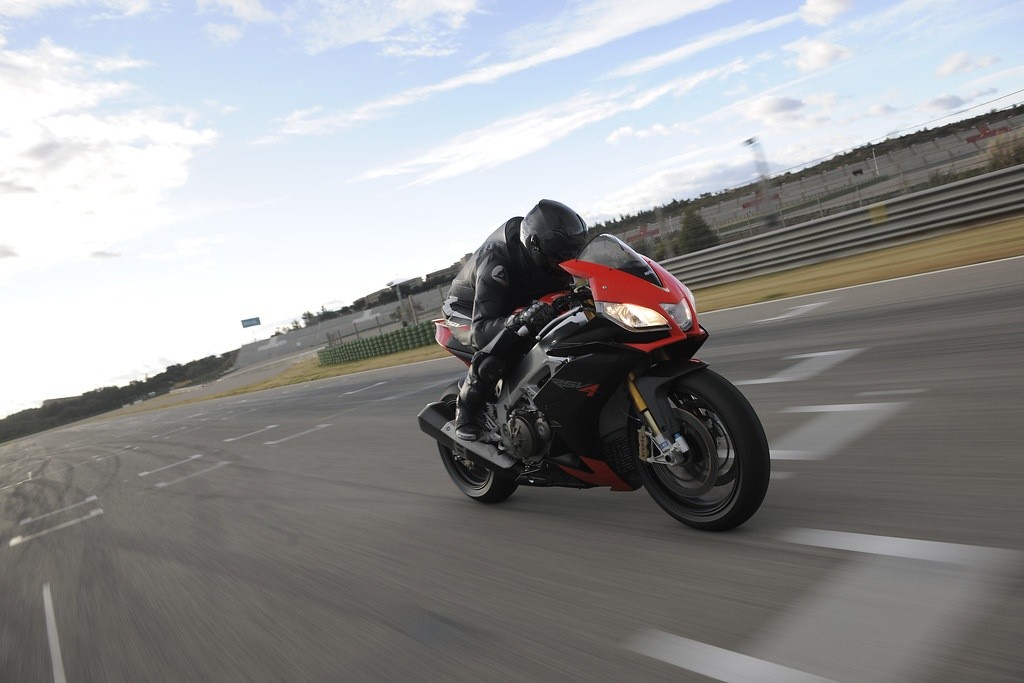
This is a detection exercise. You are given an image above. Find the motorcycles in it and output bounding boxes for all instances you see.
[416,233,771,532]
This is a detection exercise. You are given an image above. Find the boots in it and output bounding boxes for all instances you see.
[455,364,495,441]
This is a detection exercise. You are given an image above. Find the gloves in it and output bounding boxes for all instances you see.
[503,301,556,338]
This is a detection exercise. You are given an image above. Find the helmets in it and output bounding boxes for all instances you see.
[520,199,588,278]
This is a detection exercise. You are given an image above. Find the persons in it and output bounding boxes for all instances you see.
[442,199,589,441]
